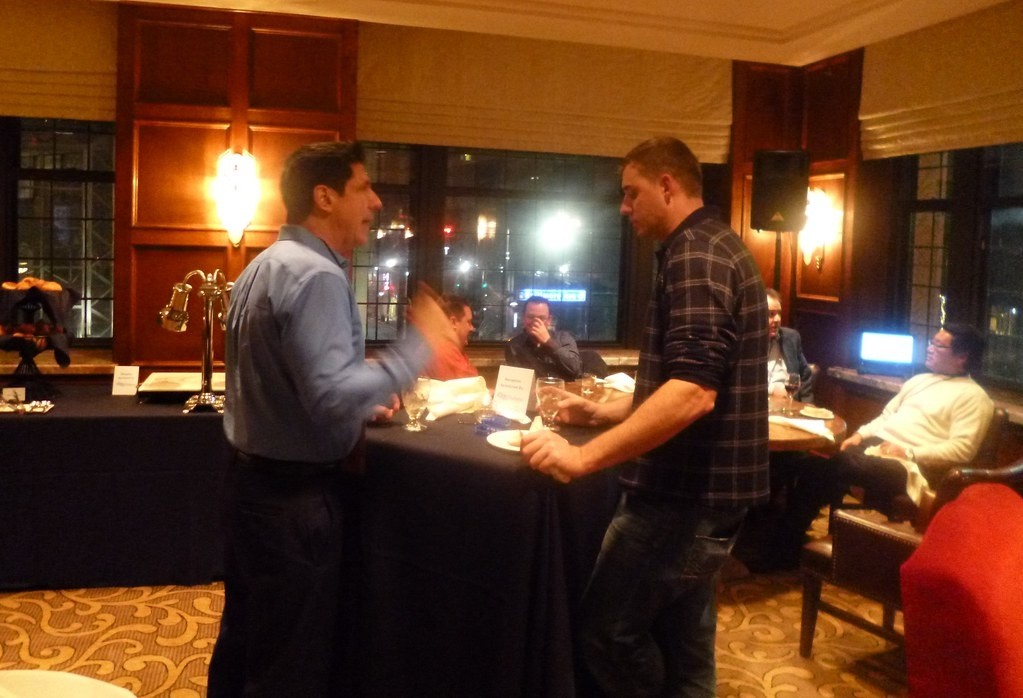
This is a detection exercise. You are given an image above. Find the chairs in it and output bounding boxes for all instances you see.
[799,408,1023,698]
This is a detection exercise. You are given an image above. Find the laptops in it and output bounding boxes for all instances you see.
[857,331,916,378]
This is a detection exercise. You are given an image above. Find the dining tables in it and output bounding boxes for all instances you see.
[556,382,849,568]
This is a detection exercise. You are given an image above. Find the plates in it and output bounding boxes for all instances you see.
[799,409,835,419]
[488,429,530,450]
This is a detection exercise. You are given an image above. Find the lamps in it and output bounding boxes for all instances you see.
[157,267,238,411]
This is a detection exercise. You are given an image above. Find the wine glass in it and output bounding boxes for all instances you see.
[401,375,430,432]
[780,373,801,416]
[536,376,566,431]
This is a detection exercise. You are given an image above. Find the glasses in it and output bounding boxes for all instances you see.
[927,339,953,352]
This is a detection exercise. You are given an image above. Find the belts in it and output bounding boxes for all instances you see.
[231,451,320,481]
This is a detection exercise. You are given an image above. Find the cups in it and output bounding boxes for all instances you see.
[580,372,597,399]
[472,409,495,434]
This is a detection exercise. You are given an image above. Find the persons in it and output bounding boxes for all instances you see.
[423,292,494,396]
[520,133,770,698]
[505,296,581,381]
[207,138,459,698]
[747,323,995,574]
[766,287,814,513]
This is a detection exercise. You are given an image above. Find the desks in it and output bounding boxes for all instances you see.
[333,387,666,698]
[0,376,244,591]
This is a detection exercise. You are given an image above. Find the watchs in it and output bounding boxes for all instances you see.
[905,448,914,462]
